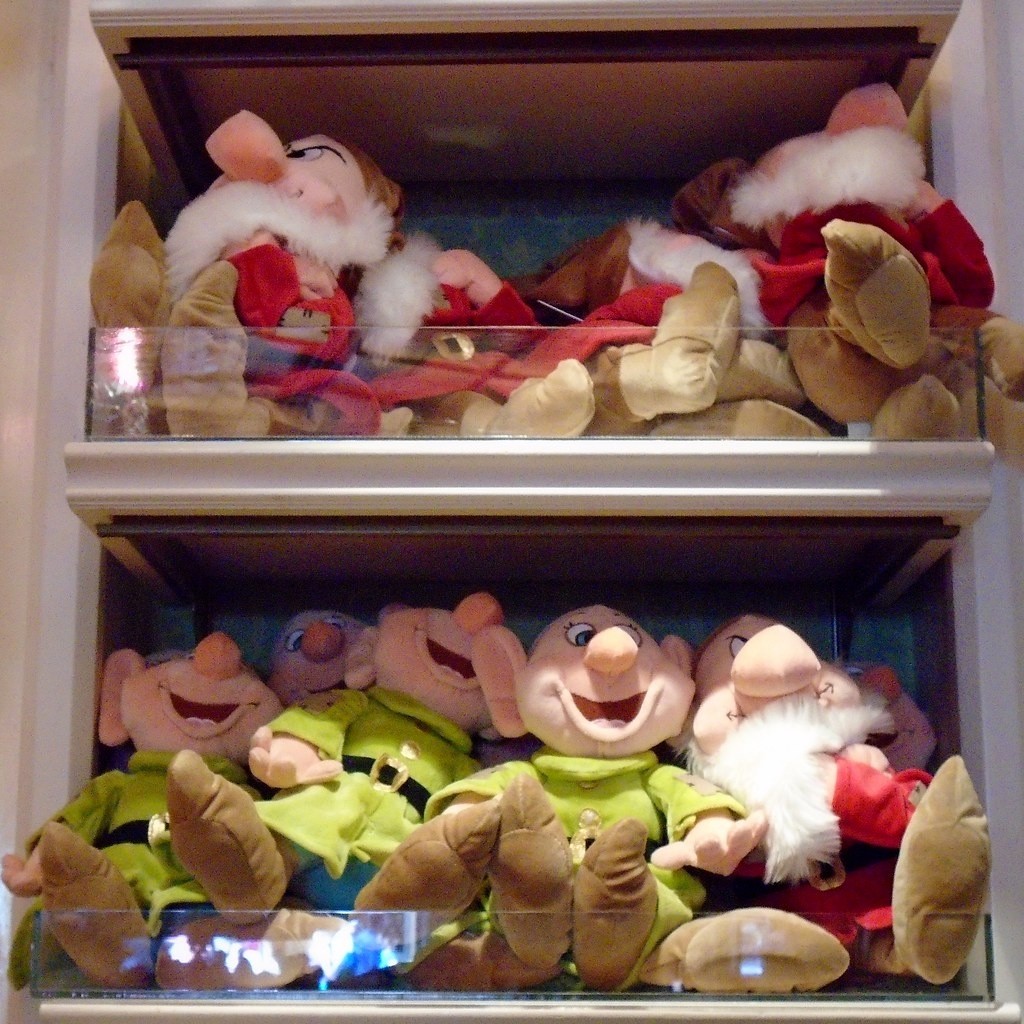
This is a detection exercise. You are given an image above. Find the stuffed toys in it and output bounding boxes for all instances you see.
[1,592,991,990]
[87,75,1023,440]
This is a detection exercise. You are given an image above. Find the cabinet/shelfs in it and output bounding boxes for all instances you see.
[1,1,1024,1024]
